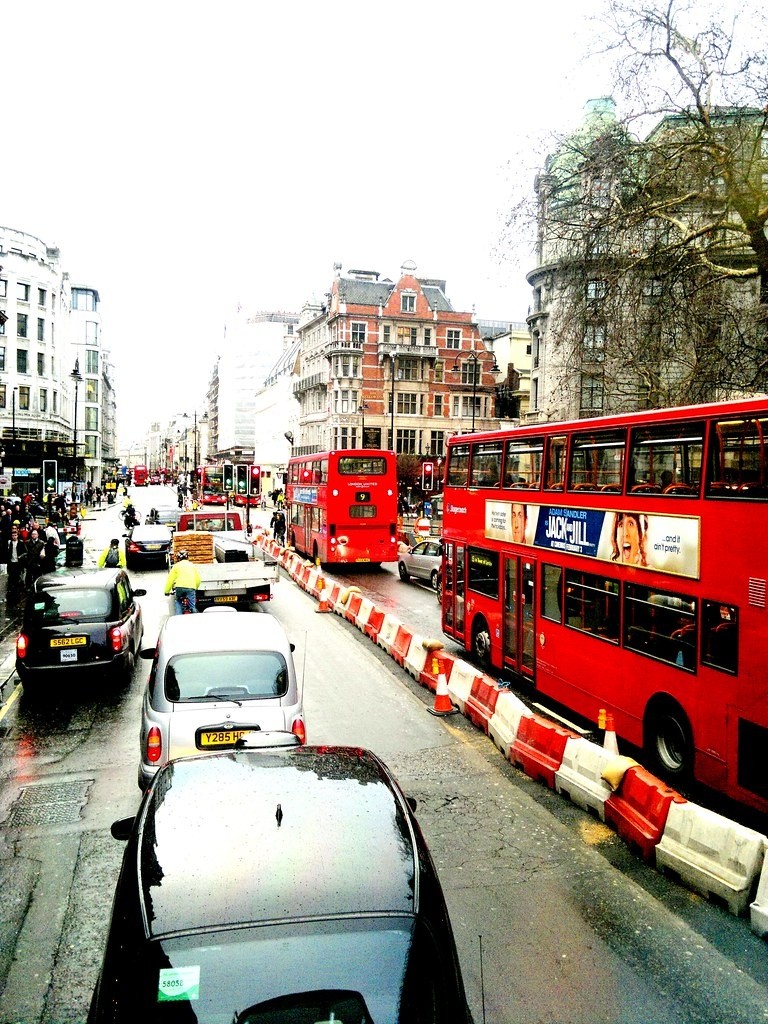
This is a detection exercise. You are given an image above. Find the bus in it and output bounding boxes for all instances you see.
[196,466,261,508]
[134,465,162,487]
[441,397,768,815]
[285,450,399,570]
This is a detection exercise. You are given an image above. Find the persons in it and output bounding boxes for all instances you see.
[164,550,201,616]
[397,497,439,521]
[156,468,197,508]
[480,460,527,488]
[511,503,528,544]
[262,488,284,510]
[670,605,738,665]
[98,539,126,569]
[270,512,286,540]
[121,504,135,529]
[219,520,234,531]
[150,508,160,525]
[609,513,650,568]
[71,471,132,507]
[0,492,78,592]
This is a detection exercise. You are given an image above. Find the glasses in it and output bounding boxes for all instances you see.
[12,528,16,529]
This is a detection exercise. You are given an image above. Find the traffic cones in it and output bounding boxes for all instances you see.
[603,713,620,756]
[312,578,333,614]
[427,661,460,716]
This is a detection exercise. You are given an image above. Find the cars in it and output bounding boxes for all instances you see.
[87,730,476,1024]
[138,606,305,788]
[16,505,183,692]
[398,540,464,590]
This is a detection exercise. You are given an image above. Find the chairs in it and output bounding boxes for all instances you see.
[463,481,762,506]
[566,586,736,670]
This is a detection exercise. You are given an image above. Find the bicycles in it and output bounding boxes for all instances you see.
[166,592,191,614]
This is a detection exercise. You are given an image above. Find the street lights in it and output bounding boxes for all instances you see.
[451,351,502,432]
[357,400,370,449]
[69,369,84,500]
[182,410,209,469]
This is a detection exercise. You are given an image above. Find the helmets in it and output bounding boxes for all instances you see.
[178,550,188,558]
[111,539,119,545]
[13,520,20,524]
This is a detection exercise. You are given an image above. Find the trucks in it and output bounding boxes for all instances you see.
[168,512,280,613]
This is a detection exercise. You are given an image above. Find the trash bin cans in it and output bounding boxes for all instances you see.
[65,536,84,567]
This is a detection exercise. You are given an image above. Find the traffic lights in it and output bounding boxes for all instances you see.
[422,462,434,490]
[223,464,261,496]
[43,459,57,494]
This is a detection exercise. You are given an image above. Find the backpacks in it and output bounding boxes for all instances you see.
[107,547,119,567]
